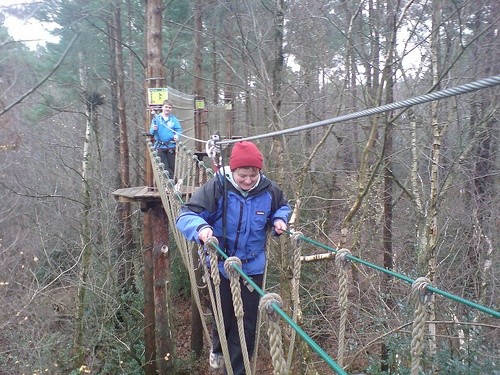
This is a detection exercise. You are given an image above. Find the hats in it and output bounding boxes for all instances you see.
[230,141,263,171]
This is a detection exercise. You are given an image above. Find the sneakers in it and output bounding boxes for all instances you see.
[209,348,224,370]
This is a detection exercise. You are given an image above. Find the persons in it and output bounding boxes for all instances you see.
[175,141,293,375]
[149,100,182,191]
